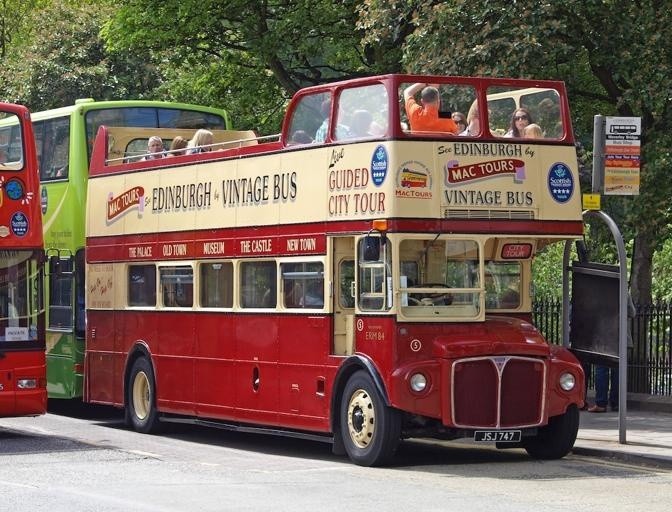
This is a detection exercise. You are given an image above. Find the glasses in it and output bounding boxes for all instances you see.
[455,120,463,125]
[513,115,529,120]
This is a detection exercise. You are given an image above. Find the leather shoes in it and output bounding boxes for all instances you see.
[589,405,608,413]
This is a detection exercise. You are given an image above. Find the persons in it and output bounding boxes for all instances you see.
[292,131,313,145]
[188,129,214,154]
[168,136,188,156]
[142,135,169,161]
[588,289,637,412]
[578,362,591,410]
[297,280,324,307]
[315,83,545,143]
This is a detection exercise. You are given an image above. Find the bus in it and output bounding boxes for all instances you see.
[82,74,586,467]
